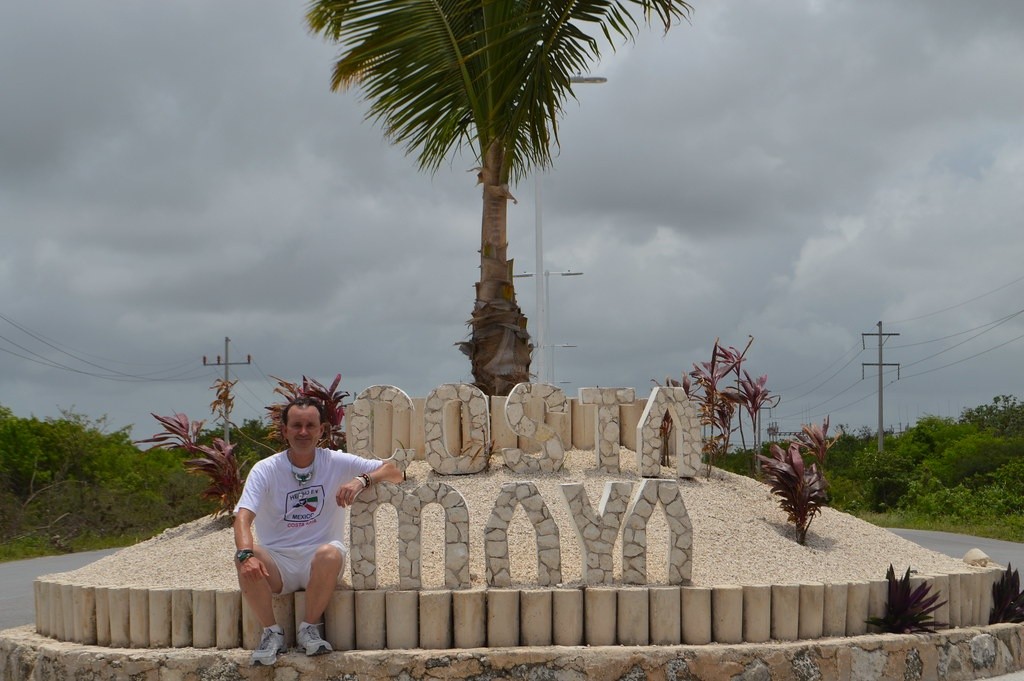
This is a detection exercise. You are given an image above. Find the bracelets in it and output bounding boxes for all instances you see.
[239,553,254,563]
[356,473,373,489]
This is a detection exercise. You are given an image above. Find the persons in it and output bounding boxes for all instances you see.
[232,397,403,666]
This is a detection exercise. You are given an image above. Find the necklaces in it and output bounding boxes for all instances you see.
[290,449,315,487]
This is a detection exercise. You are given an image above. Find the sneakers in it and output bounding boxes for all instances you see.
[295,623,332,658]
[248,628,290,665]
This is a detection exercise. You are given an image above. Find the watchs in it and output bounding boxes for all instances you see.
[236,549,253,557]
